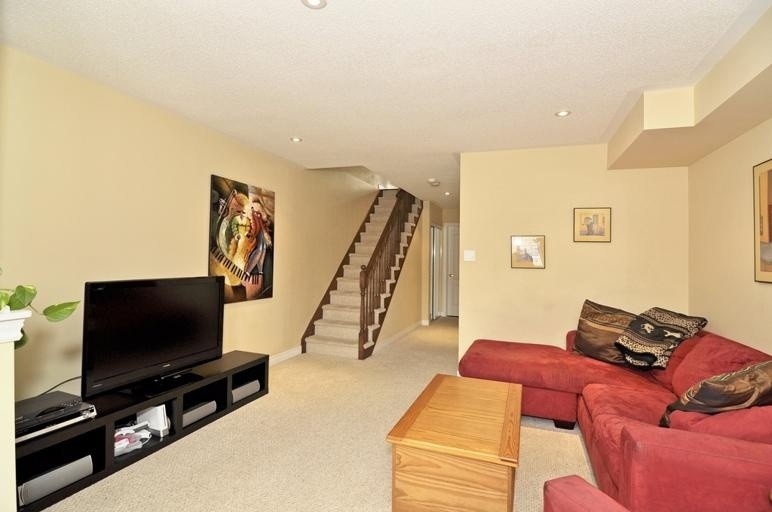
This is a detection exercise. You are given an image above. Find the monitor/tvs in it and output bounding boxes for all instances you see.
[81,275,225,400]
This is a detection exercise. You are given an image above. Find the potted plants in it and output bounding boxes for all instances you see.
[0,266,82,345]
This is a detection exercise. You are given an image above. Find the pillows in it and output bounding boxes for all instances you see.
[572,298,639,365]
[657,359,772,429]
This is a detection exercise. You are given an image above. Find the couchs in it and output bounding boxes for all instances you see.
[566,328,772,512]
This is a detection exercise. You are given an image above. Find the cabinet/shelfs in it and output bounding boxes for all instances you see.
[14,349,271,512]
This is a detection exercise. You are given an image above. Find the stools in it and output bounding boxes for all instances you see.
[458,337,581,432]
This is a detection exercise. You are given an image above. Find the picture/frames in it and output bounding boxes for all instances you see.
[572,206,612,243]
[752,158,772,284]
[510,234,546,269]
